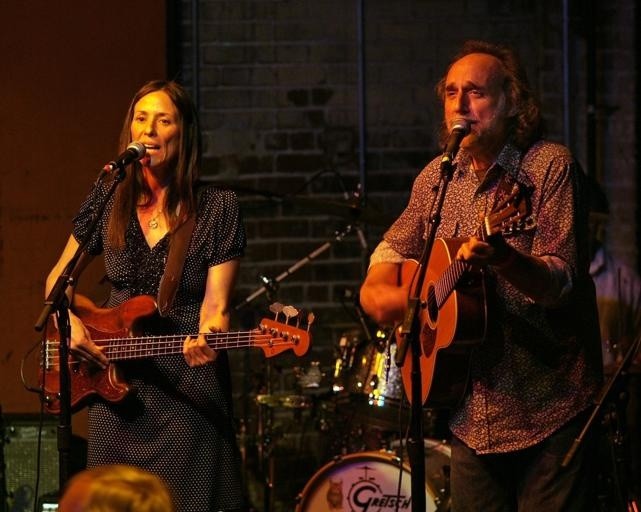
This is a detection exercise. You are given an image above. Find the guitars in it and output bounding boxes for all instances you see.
[394,187,537,411]
[40,293,315,413]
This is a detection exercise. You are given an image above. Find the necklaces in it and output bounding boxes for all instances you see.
[140,192,164,231]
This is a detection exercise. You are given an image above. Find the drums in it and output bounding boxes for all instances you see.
[332,331,409,421]
[255,344,335,413]
[296,439,453,512]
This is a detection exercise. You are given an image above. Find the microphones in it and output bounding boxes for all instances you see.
[441,118,470,167]
[335,170,368,250]
[104,143,144,172]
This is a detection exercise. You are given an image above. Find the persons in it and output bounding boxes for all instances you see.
[583,173,641,491]
[44,76,248,512]
[56,461,176,512]
[358,40,604,512]
